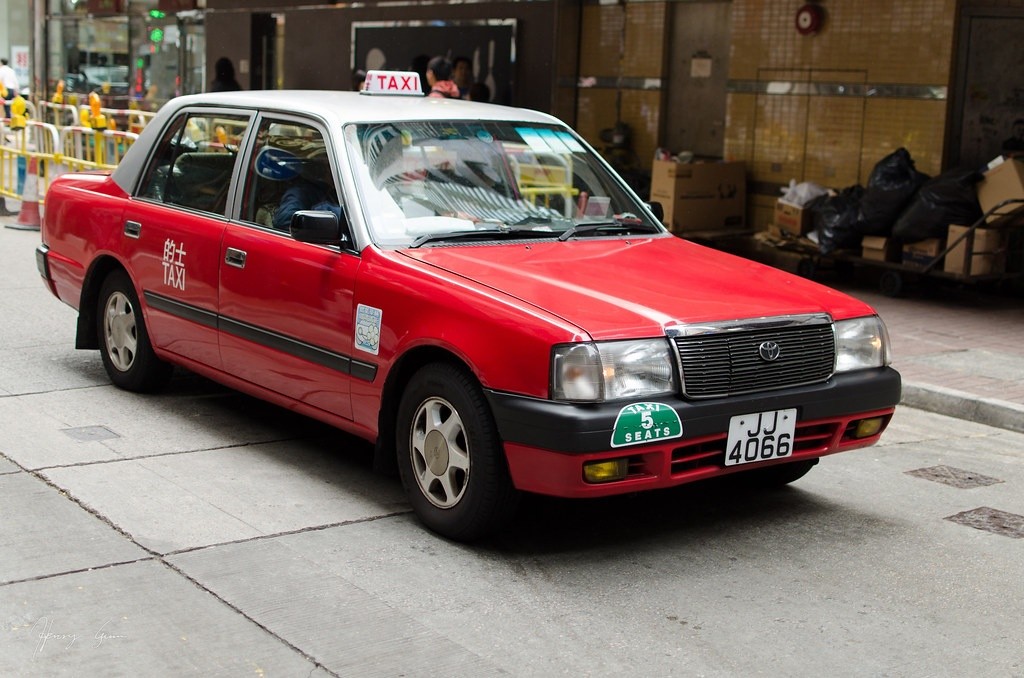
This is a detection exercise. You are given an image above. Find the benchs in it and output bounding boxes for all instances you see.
[148,152,237,216]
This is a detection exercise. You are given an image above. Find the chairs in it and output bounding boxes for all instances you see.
[254,149,335,230]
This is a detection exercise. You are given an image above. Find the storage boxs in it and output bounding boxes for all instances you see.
[944,224,1006,275]
[975,155,1024,225]
[861,236,888,262]
[649,148,748,231]
[773,200,816,237]
[903,237,940,269]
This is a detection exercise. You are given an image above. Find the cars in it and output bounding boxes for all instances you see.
[33,68,904,553]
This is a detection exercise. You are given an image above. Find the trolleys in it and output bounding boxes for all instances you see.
[757,197,1023,296]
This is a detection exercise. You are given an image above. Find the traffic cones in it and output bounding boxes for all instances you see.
[4,156,43,232]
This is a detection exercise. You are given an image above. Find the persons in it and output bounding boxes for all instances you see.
[273,151,349,246]
[352,66,367,91]
[432,134,522,224]
[411,53,433,96]
[426,54,461,100]
[469,84,492,101]
[210,57,242,92]
[449,56,477,100]
[0,58,21,127]
